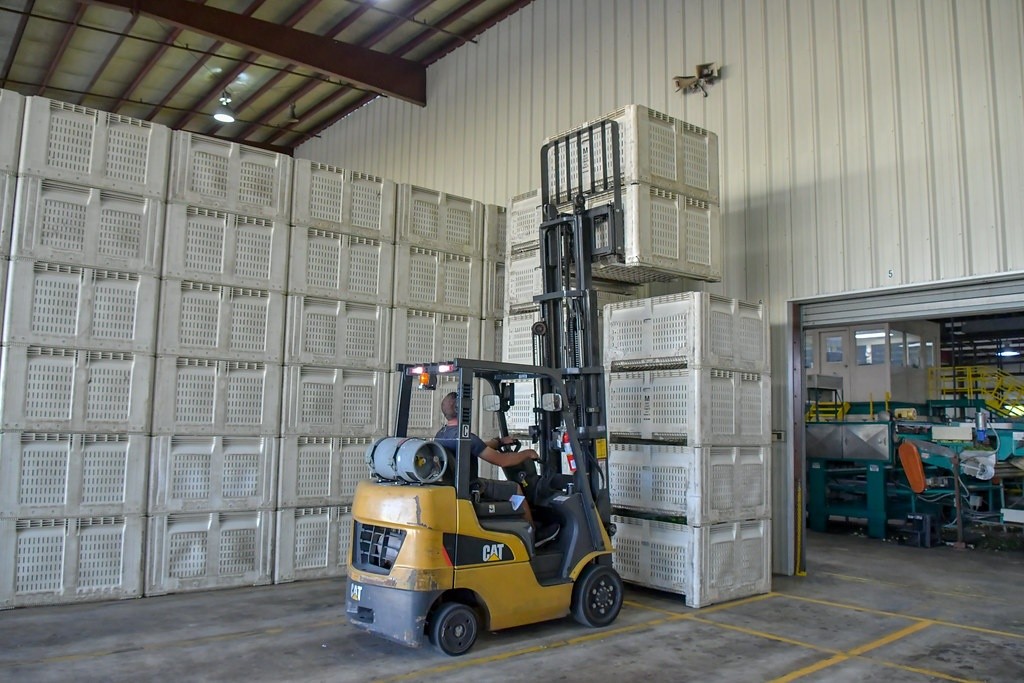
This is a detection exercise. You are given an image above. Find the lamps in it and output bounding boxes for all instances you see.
[214,89,236,123]
[288,102,299,122]
[996,341,1020,357]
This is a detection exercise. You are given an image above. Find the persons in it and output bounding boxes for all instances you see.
[433,392,560,548]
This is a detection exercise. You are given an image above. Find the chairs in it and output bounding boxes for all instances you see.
[442,447,526,516]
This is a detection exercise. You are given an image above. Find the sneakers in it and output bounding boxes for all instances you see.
[534,524,560,548]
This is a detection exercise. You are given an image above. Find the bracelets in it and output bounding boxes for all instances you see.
[493,437,501,450]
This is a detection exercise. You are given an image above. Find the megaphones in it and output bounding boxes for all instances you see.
[672,75,696,91]
[697,62,718,81]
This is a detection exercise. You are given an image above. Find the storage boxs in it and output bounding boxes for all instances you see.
[499,189,636,481]
[547,103,724,286]
[0,88,505,610]
[602,292,771,609]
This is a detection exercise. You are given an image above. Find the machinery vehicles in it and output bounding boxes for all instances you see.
[342,118,646,658]
